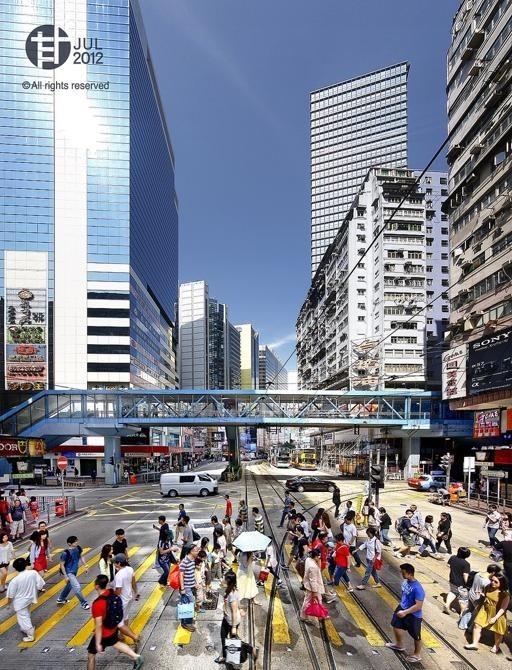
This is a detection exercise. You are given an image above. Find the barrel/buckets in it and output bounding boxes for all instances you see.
[405,654,422,663]
[464,643,479,650]
[252,601,263,606]
[490,644,500,653]
[385,643,406,651]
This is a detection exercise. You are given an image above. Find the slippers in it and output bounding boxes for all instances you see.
[59,545,82,576]
[93,588,123,630]
[169,560,180,590]
[311,519,320,531]
[395,516,411,537]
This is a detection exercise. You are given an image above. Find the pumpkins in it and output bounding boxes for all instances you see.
[64,482,81,488]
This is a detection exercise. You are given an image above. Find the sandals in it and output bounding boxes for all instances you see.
[267,445,291,468]
[290,447,317,472]
[221,444,244,460]
[244,450,261,460]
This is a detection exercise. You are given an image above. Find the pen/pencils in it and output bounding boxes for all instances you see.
[34,464,49,475]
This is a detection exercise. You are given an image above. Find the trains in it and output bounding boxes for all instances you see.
[57,455,68,470]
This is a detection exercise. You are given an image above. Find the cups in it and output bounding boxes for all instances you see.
[491,579,498,584]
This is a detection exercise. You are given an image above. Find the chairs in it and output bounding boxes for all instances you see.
[254,565,307,592]
[22,634,35,642]
[197,585,219,613]
[0,558,53,592]
[326,560,382,604]
[133,635,144,653]
[131,655,145,670]
[10,520,37,542]
[253,646,259,659]
[393,551,454,615]
[56,599,70,604]
[214,656,226,663]
[181,623,196,632]
[80,601,91,610]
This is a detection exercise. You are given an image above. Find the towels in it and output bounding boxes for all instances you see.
[130,472,137,484]
[54,499,68,516]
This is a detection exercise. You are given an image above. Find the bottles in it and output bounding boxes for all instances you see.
[110,553,127,564]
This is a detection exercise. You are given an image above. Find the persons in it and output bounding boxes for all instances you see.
[123,469,128,483]
[43,466,78,479]
[91,469,98,483]
[278,487,512,663]
[120,404,404,418]
[129,453,195,472]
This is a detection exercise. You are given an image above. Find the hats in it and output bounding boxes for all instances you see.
[286,474,337,493]
[407,470,467,493]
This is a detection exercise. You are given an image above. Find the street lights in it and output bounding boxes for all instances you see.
[304,596,329,618]
[331,551,336,558]
[192,529,201,541]
[417,523,431,539]
[167,541,180,564]
[259,567,270,581]
[177,594,195,620]
[225,633,252,665]
[373,559,384,570]
[437,534,448,541]
[25,554,31,566]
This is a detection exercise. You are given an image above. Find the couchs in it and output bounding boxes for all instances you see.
[229,450,234,461]
[109,456,115,465]
[449,455,454,464]
[442,457,450,471]
[369,463,384,489]
[438,455,446,470]
